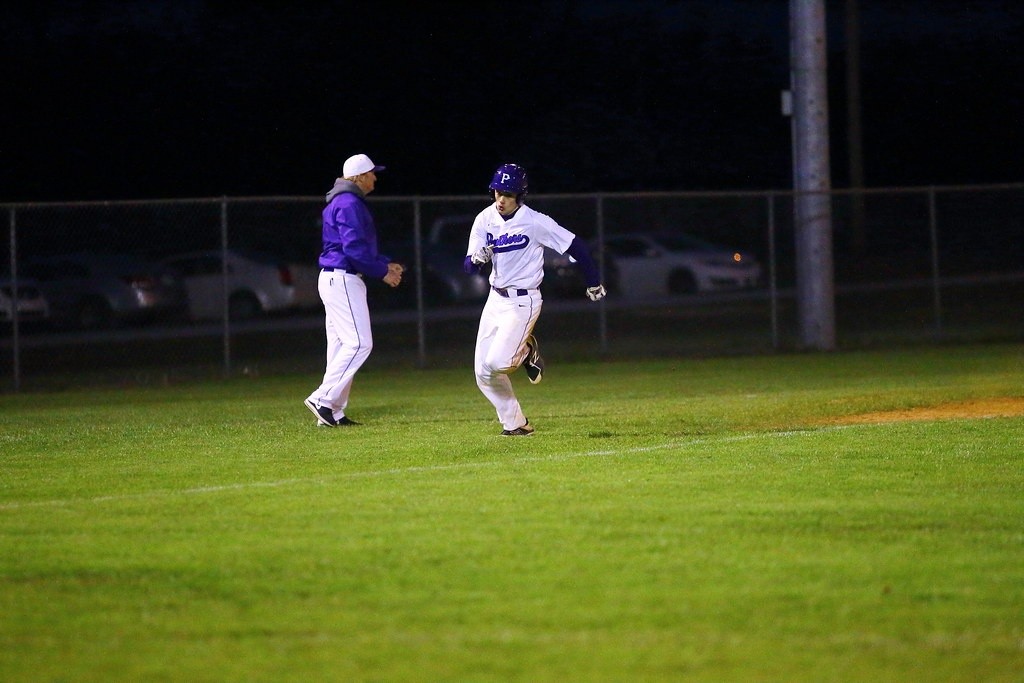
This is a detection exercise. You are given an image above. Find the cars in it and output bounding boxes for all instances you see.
[1,265,185,325]
[591,232,782,300]
[446,248,586,303]
[173,250,322,320]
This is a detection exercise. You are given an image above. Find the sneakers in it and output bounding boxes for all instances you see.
[499,417,535,436]
[304,398,365,428]
[522,334,544,385]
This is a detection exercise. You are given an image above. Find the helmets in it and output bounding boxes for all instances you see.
[489,164,529,206]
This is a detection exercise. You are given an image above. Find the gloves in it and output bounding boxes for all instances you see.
[586,283,607,302]
[471,244,494,266]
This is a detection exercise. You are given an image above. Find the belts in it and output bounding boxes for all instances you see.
[492,285,540,298]
[323,267,357,275]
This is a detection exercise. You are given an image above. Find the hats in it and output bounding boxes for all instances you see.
[343,154,385,179]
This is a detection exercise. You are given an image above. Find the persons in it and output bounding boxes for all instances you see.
[304,153,403,427]
[463,163,608,437]
